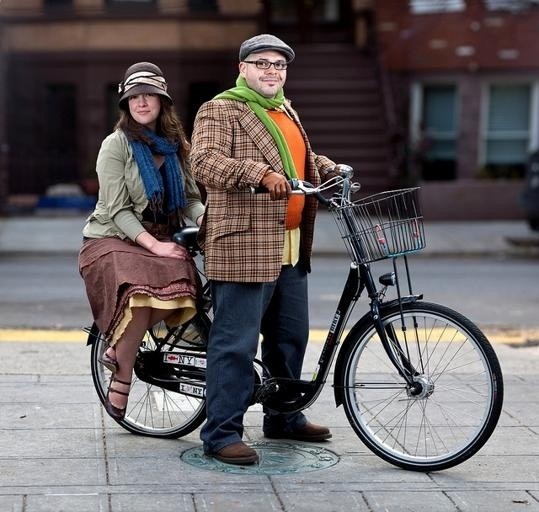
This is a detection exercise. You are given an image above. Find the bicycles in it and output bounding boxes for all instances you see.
[82,165,506,474]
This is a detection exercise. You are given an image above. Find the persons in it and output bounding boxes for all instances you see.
[77,62,206,420]
[186,32,343,467]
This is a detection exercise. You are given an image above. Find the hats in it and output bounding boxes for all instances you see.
[240,35,295,64]
[118,62,173,109]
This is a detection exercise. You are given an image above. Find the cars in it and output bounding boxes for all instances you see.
[520,148,539,235]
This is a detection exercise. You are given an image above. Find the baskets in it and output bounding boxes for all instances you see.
[330,187,427,265]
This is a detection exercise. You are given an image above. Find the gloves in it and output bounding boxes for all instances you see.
[262,169,291,201]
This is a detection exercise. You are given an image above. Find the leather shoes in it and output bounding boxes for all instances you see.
[99,347,118,373]
[105,373,132,420]
[265,420,332,441]
[215,442,258,463]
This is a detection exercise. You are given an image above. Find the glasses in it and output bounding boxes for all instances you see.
[244,60,287,71]
